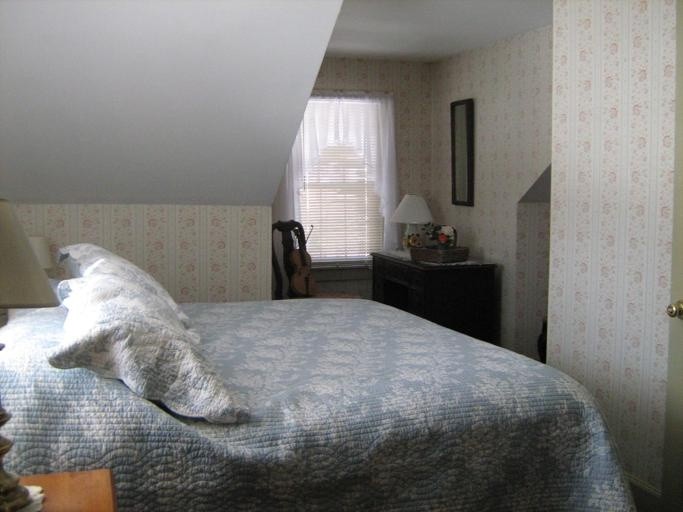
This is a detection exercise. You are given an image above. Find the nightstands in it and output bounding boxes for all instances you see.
[14,466,121,511]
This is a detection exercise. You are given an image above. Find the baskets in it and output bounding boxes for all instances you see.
[410,224,468,262]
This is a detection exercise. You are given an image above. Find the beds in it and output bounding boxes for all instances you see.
[0,295,635,512]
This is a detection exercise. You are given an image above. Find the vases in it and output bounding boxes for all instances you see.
[409,248,469,263]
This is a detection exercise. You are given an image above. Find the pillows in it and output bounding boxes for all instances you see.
[56,241,194,331]
[46,275,254,426]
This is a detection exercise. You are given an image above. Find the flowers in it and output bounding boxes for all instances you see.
[402,220,460,248]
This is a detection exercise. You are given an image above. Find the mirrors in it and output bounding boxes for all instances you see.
[448,97,474,207]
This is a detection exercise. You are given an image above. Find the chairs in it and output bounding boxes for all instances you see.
[270,216,310,299]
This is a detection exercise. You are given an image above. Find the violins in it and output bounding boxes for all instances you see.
[285,228,314,297]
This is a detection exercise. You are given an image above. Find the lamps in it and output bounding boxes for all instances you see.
[23,234,57,272]
[0,196,61,512]
[390,190,437,252]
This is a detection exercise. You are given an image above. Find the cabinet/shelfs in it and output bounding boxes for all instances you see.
[369,250,504,348]
